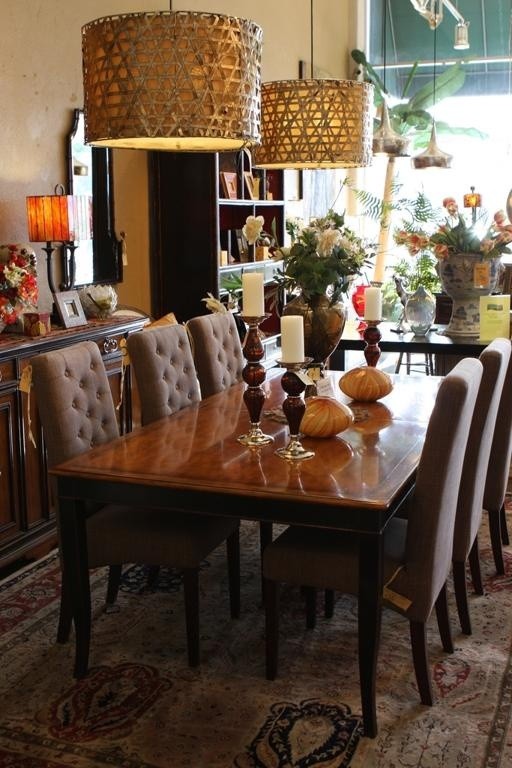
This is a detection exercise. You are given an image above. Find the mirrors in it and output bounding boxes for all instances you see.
[67,104,124,287]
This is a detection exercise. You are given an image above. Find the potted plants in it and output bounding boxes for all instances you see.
[402,256,436,335]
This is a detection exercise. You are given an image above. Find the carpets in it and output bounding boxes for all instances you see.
[3,488,506,764]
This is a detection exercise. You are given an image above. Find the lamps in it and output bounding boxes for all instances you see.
[26,183,76,296]
[410,0,469,51]
[68,2,262,163]
[252,75,374,170]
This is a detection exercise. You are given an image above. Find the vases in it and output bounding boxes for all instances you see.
[441,247,500,334]
[282,287,359,361]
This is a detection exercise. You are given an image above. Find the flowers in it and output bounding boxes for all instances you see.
[2,243,43,330]
[288,211,376,293]
[84,274,116,322]
[400,193,512,256]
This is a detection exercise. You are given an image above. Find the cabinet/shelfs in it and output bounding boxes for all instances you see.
[165,155,286,312]
[0,312,151,576]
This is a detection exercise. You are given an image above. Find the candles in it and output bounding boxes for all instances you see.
[281,315,304,363]
[242,273,265,317]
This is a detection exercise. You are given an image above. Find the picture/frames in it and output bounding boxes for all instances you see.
[54,290,87,329]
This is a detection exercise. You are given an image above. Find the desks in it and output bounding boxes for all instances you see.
[40,369,452,736]
[260,313,508,372]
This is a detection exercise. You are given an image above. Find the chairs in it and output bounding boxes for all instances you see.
[323,337,512,637]
[123,326,206,432]
[185,309,251,403]
[30,340,239,669]
[259,356,482,707]
[482,358,512,573]
